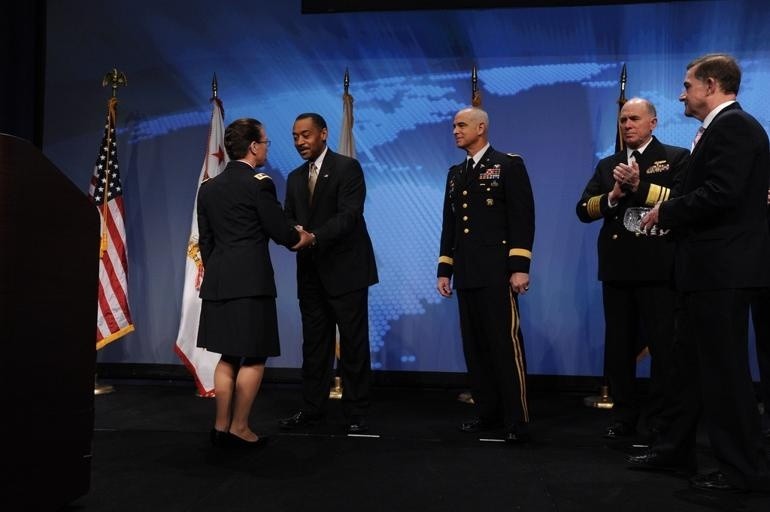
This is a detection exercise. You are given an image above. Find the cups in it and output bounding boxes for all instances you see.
[623,206,669,236]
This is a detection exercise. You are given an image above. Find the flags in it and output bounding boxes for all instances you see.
[615,96,647,366]
[89,98,136,352]
[332,94,355,372]
[470,86,484,111]
[173,96,232,399]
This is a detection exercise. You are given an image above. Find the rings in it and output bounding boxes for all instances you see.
[620,175,625,180]
[526,287,529,291]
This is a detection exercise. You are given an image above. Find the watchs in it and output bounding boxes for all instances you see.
[309,239,317,250]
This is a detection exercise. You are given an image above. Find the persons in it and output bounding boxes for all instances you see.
[275,112,379,433]
[196,118,312,454]
[436,106,536,449]
[621,56,770,498]
[574,96,690,456]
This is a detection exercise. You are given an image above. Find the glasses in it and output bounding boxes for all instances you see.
[259,140,271,147]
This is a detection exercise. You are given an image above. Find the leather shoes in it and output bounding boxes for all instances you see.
[602,421,631,440]
[506,424,529,442]
[623,444,656,469]
[347,415,370,433]
[222,432,270,450]
[279,408,327,429]
[688,470,748,495]
[456,419,483,433]
[211,427,228,448]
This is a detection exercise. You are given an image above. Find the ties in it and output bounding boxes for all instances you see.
[466,158,475,180]
[308,163,318,209]
[630,150,641,160]
